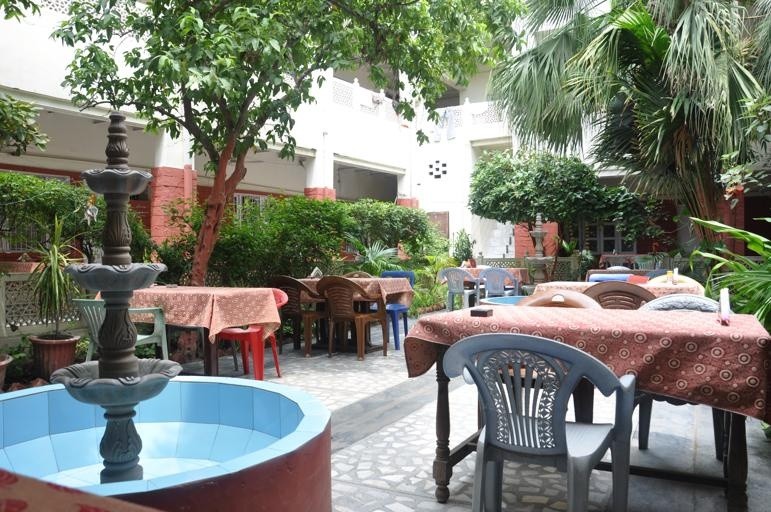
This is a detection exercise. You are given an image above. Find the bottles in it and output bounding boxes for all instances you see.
[667,271,674,285]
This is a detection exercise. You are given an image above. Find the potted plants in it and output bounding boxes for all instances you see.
[16,206,88,375]
